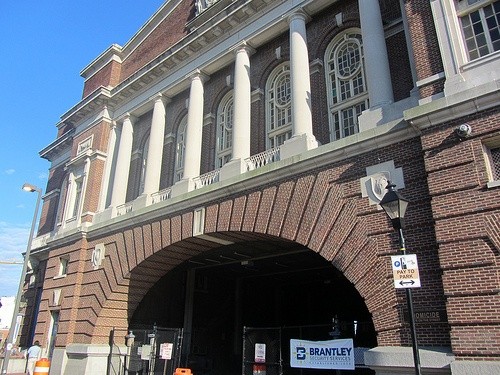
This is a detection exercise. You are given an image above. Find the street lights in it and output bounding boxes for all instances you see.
[378,179,423,375]
[1,184,43,375]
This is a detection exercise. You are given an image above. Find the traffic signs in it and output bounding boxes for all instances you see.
[390,253,421,289]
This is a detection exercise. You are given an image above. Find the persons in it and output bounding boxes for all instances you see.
[11,345,26,357]
[27,341,42,375]
